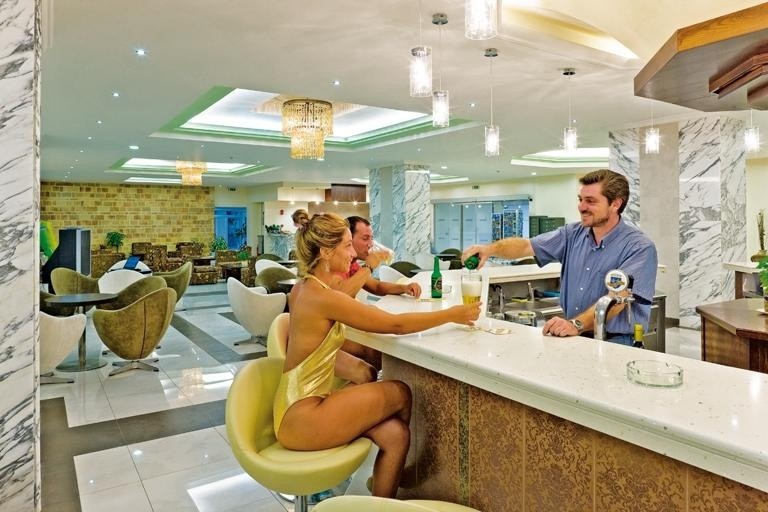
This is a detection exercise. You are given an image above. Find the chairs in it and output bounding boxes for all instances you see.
[225,357,373,512]
[308,494,480,512]
[266,312,352,504]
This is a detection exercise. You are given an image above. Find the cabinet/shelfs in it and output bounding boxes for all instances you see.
[433,202,463,255]
[462,202,493,256]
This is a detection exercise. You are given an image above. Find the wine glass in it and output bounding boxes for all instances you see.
[461,273,482,331]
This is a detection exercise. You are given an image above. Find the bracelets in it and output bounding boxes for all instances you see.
[360,264,374,274]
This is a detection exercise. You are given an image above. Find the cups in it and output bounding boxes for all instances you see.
[483,310,506,334]
[368,242,392,265]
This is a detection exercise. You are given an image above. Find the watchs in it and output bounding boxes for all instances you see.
[569,319,584,335]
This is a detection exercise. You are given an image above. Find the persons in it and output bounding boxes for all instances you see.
[329,217,423,385]
[272,209,482,499]
[461,168,658,346]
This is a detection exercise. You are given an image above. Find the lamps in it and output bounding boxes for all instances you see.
[561,67,578,160]
[409,0,433,99]
[281,97,333,161]
[744,108,761,154]
[482,48,501,156]
[464,0,499,40]
[431,13,451,127]
[644,97,661,154]
[174,159,207,187]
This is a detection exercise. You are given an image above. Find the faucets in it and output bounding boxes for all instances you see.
[593,292,636,340]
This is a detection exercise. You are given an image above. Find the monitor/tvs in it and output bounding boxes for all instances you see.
[41,228,92,294]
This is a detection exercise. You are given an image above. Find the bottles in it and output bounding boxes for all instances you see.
[429,256,443,299]
[630,322,646,349]
[463,256,481,270]
